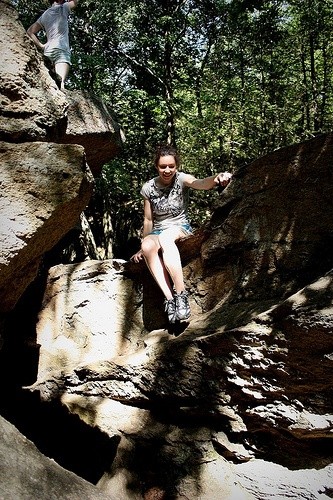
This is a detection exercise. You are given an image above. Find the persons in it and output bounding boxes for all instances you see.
[128,146,232,323]
[27,0,79,93]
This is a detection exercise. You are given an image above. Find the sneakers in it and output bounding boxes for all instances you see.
[173,290,191,322]
[162,298,175,323]
